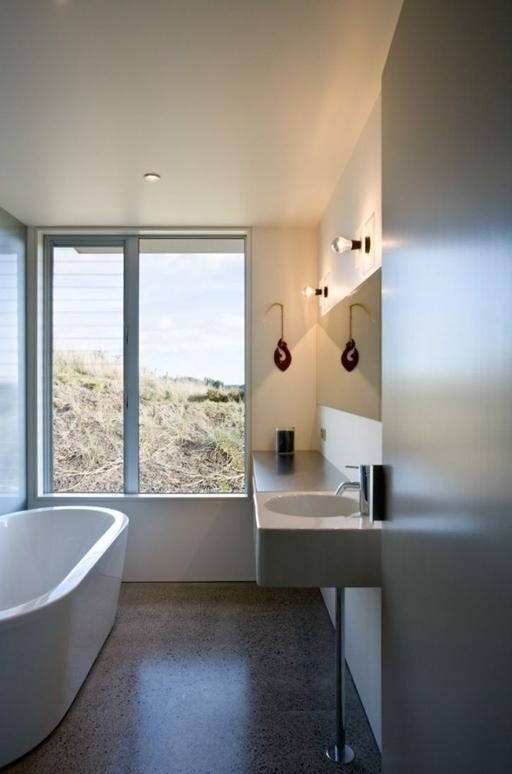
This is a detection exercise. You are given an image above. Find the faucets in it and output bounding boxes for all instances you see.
[333,464,360,495]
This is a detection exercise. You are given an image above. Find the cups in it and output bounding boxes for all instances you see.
[276,427,294,455]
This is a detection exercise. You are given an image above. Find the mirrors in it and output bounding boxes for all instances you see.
[316,266,381,422]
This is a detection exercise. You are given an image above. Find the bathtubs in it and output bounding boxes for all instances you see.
[0,505,129,767]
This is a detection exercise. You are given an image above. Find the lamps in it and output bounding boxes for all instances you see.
[331,236,361,254]
[301,286,322,298]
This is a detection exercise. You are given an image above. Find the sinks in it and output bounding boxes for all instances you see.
[260,488,362,519]
